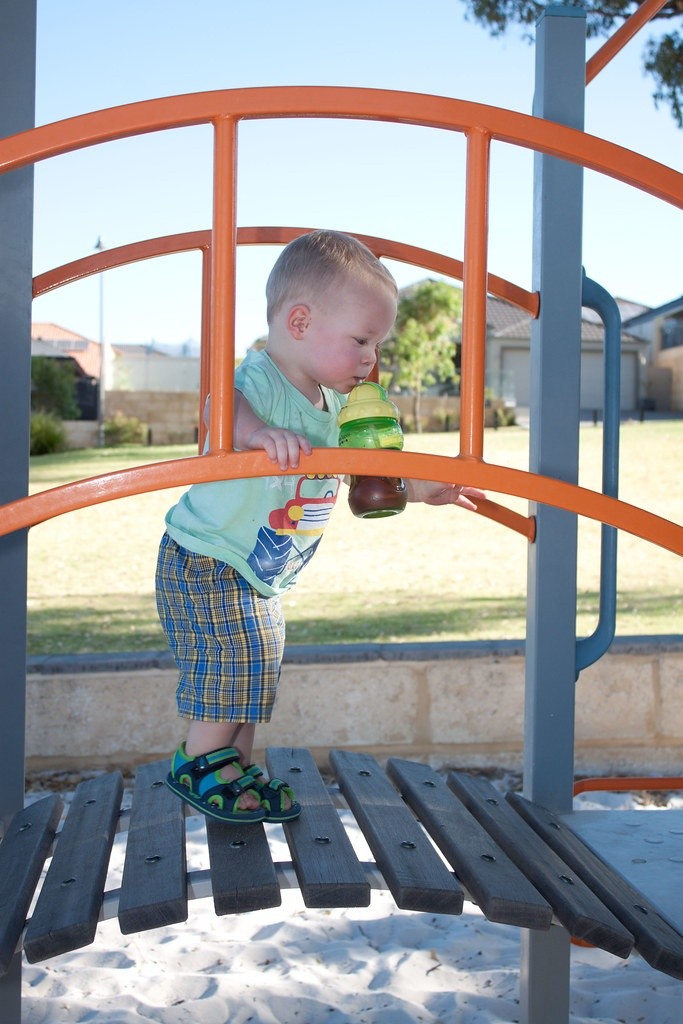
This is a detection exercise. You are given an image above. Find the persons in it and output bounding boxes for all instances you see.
[149,227,487,825]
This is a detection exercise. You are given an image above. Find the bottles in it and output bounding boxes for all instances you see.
[338,379,407,519]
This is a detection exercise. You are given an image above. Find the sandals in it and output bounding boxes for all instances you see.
[165,740,267,826]
[243,764,302,823]
